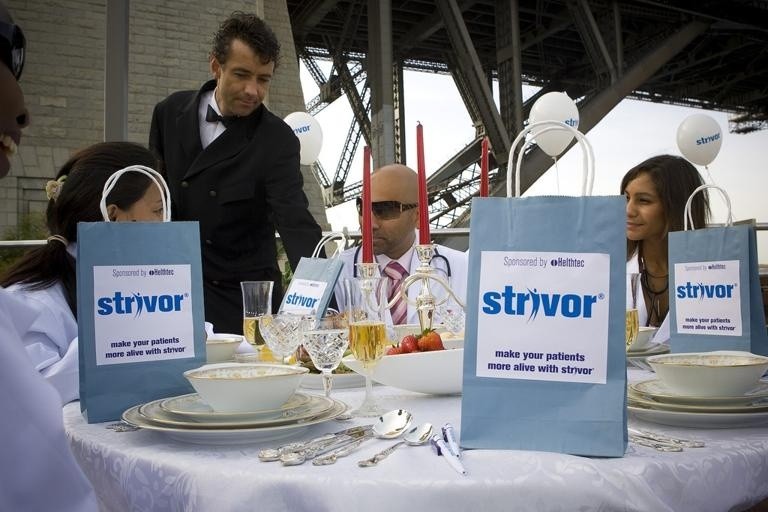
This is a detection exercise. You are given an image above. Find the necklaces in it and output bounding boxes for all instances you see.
[639,244,670,327]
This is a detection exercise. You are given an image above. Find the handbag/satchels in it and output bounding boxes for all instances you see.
[456,118,634,464]
[666,183,765,364]
[74,163,207,426]
[277,231,349,327]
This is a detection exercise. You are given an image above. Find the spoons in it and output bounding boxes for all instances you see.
[309,407,413,466]
[358,423,437,468]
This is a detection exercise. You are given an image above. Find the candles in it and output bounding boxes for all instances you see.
[479,133,491,198]
[415,122,432,246]
[362,143,375,265]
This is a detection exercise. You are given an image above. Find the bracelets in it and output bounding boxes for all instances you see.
[325,308,335,317]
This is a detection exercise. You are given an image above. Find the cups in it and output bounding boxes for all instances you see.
[626,270,643,351]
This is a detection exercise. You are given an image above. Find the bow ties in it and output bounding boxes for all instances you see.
[202,104,241,128]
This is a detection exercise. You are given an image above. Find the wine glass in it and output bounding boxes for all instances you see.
[239,279,277,352]
[256,315,307,365]
[351,277,389,417]
[297,317,350,398]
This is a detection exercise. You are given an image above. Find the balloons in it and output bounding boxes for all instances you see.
[677,113,723,166]
[282,112,323,166]
[528,91,580,158]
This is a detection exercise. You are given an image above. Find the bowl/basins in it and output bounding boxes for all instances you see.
[204,335,242,361]
[344,340,465,398]
[184,364,310,410]
[629,325,656,349]
[646,350,762,396]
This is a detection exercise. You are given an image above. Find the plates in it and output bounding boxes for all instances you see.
[625,343,671,368]
[293,370,378,388]
[624,380,766,430]
[121,393,349,446]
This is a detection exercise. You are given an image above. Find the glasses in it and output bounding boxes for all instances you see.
[0,22,26,78]
[354,195,421,218]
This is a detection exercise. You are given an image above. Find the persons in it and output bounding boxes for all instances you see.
[1,5,109,511]
[148,8,345,334]
[0,141,169,409]
[620,153,710,343]
[328,164,468,331]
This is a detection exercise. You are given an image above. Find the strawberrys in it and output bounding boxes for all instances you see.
[389,330,445,355]
